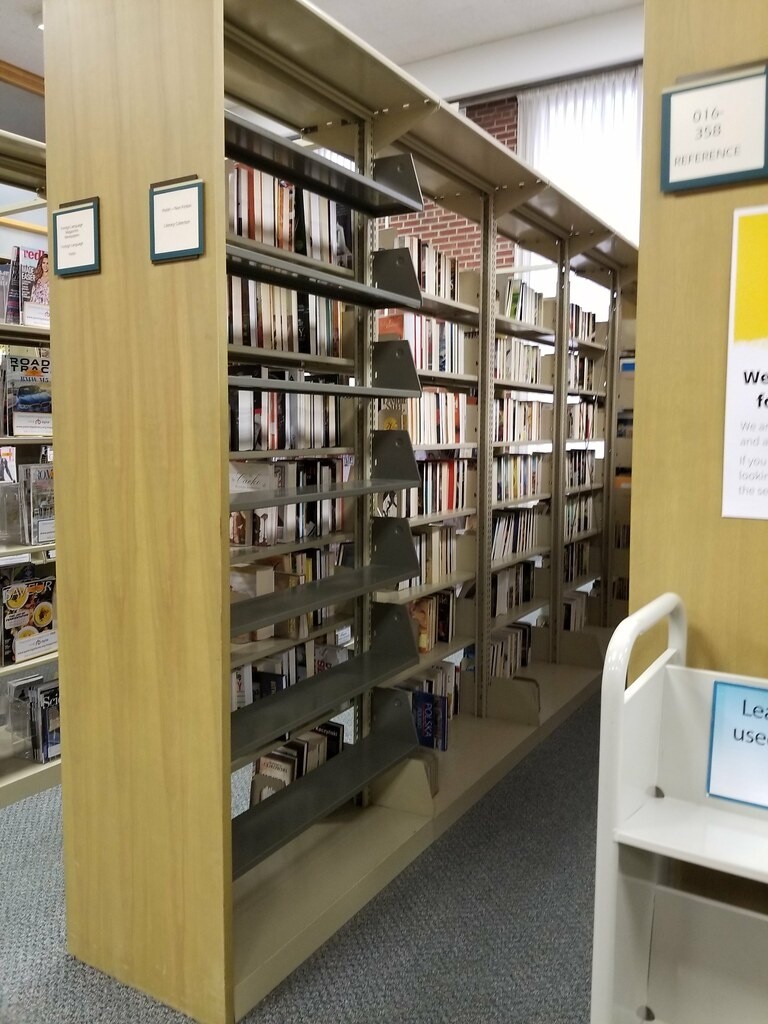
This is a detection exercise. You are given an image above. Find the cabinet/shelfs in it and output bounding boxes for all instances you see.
[39,5,642,1022]
[0,128,63,814]
[627,0,768,746]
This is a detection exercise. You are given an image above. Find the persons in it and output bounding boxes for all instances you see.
[30,254,48,304]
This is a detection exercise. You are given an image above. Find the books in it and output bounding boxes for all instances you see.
[0,245,62,764]
[228,161,608,802]
